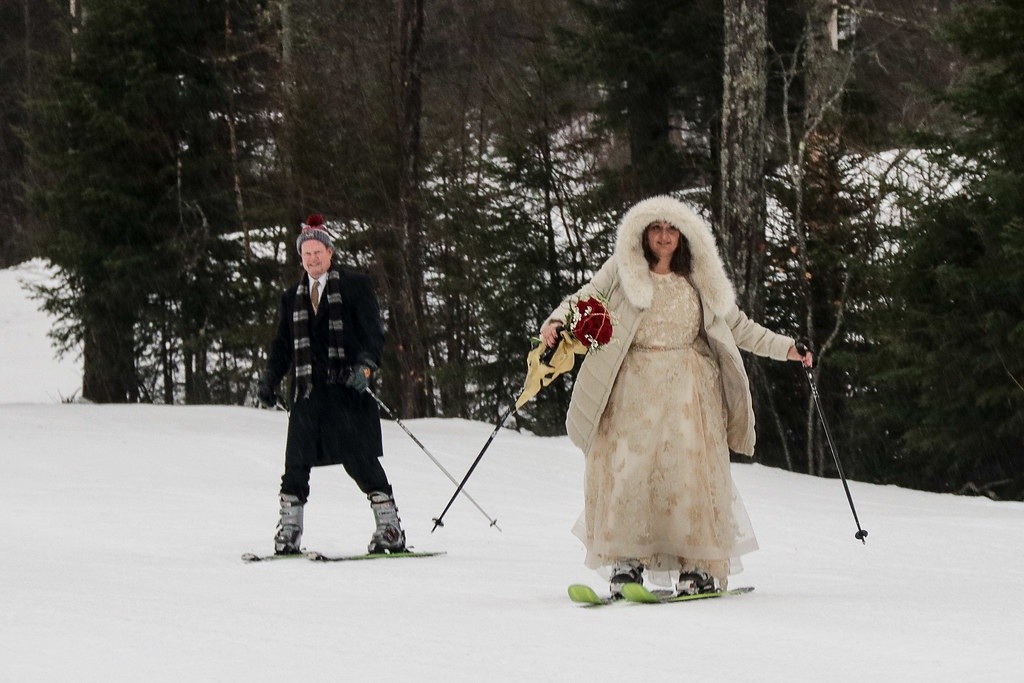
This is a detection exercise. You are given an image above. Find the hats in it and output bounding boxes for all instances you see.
[297,213,334,256]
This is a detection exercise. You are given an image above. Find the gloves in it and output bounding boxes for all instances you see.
[257,378,277,407]
[338,360,371,394]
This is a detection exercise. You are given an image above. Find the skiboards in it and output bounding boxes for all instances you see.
[565,580,756,606]
[241,549,450,565]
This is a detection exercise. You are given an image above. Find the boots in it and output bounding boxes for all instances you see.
[275,493,304,553]
[367,485,404,552]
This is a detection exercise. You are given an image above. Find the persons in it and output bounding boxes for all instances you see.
[256,213,407,553]
[538,195,812,596]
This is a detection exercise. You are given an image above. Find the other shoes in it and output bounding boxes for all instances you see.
[609,558,644,593]
[676,563,714,593]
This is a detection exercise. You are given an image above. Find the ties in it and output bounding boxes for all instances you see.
[311,281,320,310]
